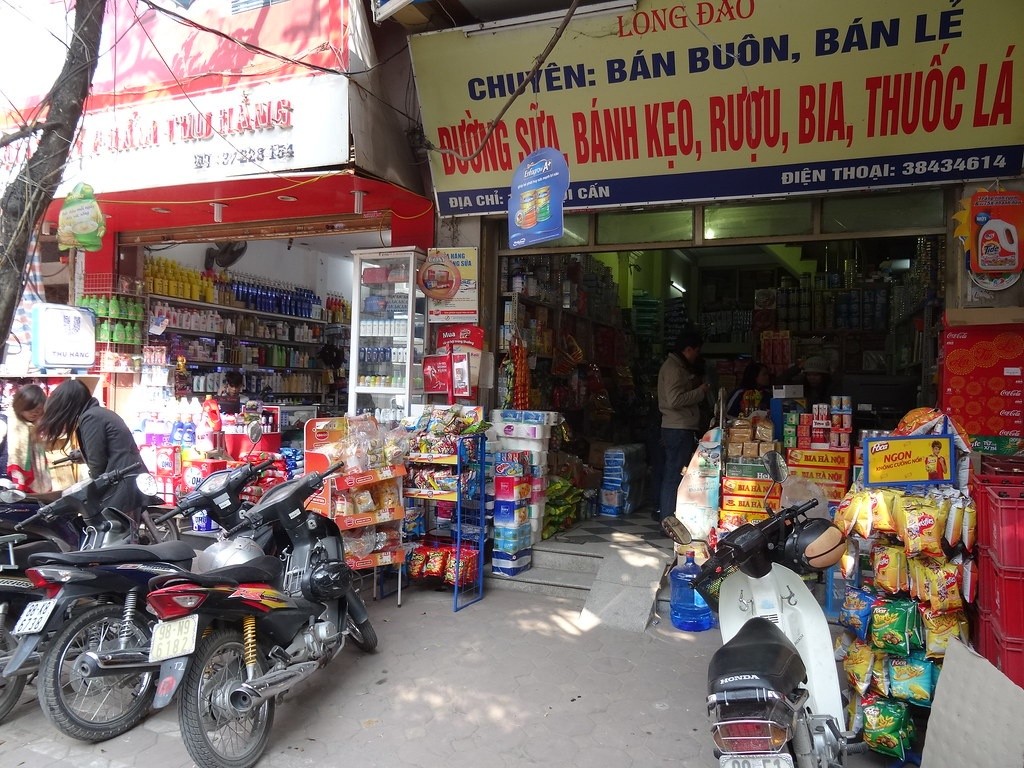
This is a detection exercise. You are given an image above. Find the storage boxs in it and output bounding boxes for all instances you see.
[719,423,1024,690]
[140,429,282,503]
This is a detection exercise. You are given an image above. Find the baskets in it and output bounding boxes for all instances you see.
[690,556,739,613]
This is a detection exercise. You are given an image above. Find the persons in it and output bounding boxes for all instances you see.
[726,361,777,416]
[652,329,713,526]
[32,379,157,551]
[216,368,249,405]
[799,356,838,407]
[0,383,53,496]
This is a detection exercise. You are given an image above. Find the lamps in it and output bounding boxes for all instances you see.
[209,202,228,222]
[350,190,368,215]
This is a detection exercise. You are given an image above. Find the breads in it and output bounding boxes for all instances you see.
[727,418,776,457]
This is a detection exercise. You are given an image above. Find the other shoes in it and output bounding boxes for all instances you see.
[651,509,660,520]
[659,522,669,537]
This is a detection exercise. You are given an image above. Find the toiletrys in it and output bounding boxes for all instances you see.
[356,317,424,424]
[143,254,322,394]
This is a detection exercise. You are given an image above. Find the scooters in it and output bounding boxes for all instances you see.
[661,450,857,768]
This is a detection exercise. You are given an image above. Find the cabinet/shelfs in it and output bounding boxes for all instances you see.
[304,417,483,613]
[497,282,945,448]
[349,248,421,416]
[0,373,106,491]
[84,290,327,402]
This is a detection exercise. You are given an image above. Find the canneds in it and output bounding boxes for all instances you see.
[360,375,392,386]
[776,271,888,332]
[359,346,391,362]
[831,396,852,447]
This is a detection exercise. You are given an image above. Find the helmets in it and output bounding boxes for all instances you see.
[784,518,846,573]
[301,559,354,603]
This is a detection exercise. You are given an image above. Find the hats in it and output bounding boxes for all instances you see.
[225,371,242,387]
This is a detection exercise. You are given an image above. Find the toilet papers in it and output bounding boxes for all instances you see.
[491,409,558,548]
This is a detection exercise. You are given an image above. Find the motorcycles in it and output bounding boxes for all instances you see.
[3,420,278,744]
[1,462,152,721]
[0,447,181,572]
[146,460,379,768]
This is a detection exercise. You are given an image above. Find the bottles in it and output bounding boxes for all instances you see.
[670,551,712,631]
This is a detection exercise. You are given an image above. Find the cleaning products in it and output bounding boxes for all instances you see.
[75,293,145,345]
[978,207,1018,270]
[138,395,227,508]
[220,405,273,434]
